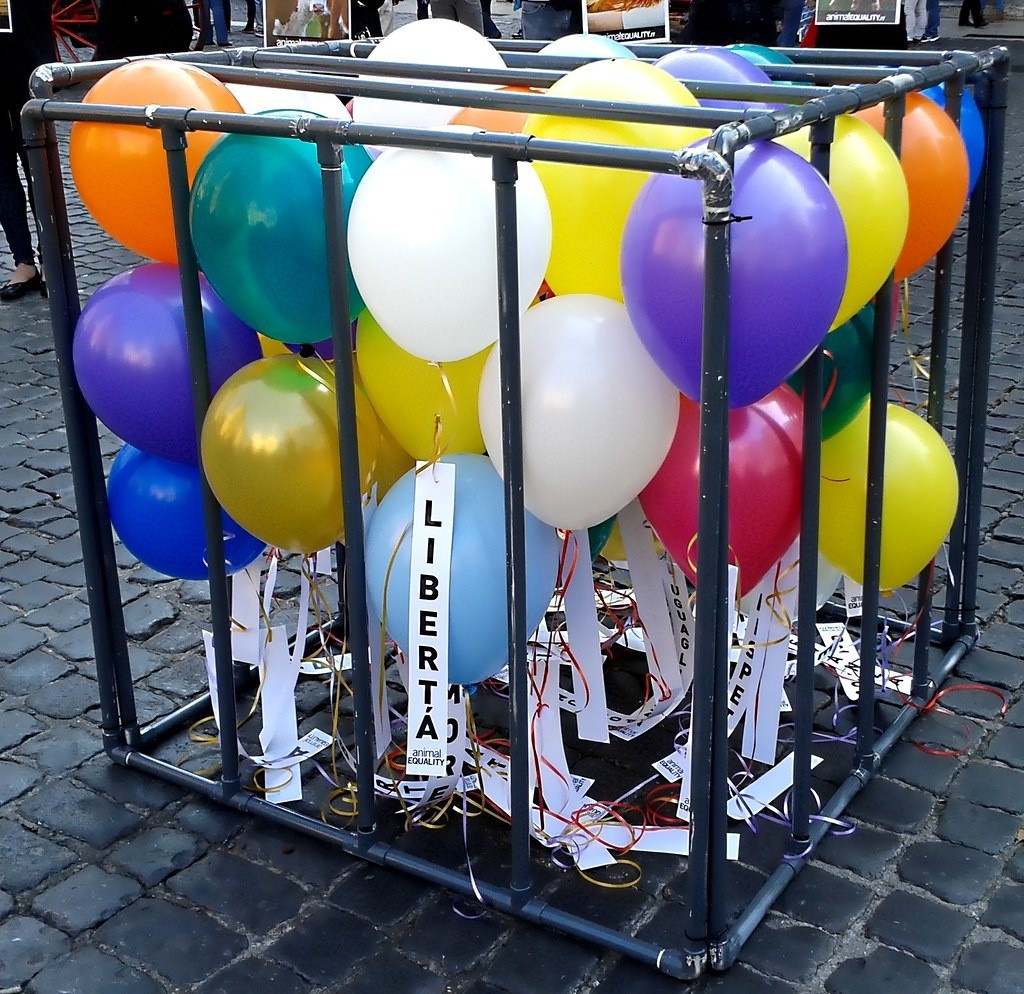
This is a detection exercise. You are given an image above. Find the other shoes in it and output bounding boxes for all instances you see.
[204,41,217,47]
[958,19,973,26]
[39,266,48,297]
[218,42,233,48]
[993,12,1004,19]
[974,21,989,28]
[0,265,41,301]
[920,33,939,42]
[512,29,523,39]
[255,25,264,38]
[241,28,254,33]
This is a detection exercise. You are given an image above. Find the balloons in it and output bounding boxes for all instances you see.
[75,17,988,688]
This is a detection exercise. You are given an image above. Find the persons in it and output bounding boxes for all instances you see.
[0,0,1006,302]
